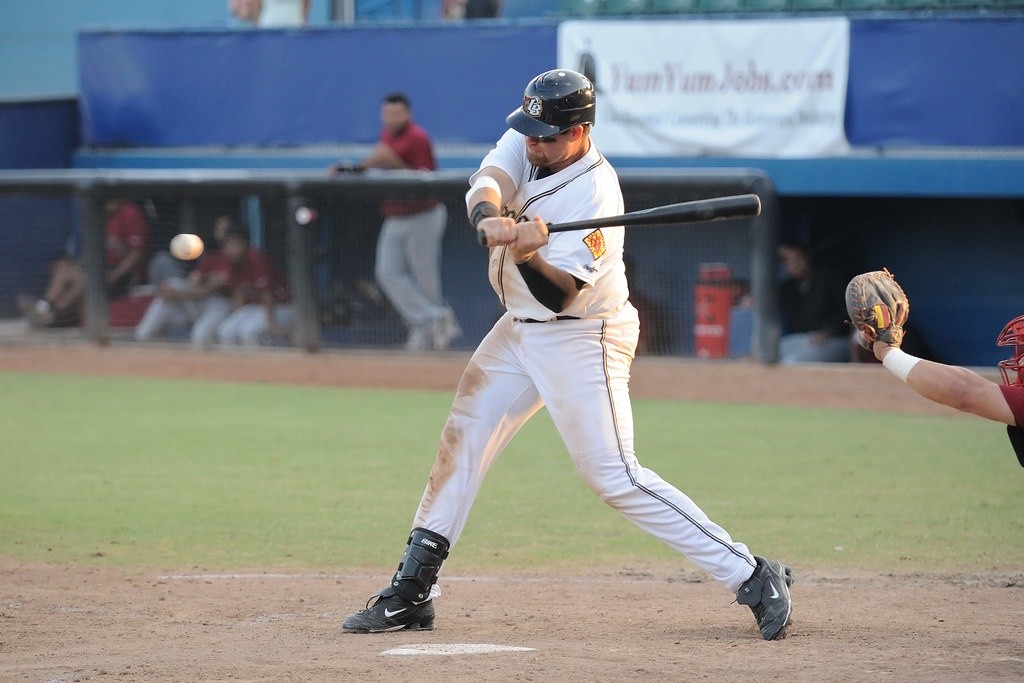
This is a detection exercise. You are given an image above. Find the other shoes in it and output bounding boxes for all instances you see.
[36,297,59,327]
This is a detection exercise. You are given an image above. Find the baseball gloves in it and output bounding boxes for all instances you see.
[843,266,913,349]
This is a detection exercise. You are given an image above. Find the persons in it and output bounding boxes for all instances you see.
[132,212,292,348]
[441,0,500,19]
[19,190,147,327]
[339,69,794,640]
[328,94,461,350]
[742,240,851,364]
[845,269,1024,467]
[230,0,307,26]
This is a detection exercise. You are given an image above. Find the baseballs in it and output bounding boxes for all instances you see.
[169,233,205,261]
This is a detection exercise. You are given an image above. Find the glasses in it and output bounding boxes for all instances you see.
[536,137,557,143]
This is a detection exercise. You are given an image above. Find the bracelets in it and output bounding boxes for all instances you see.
[883,349,920,384]
[470,201,499,229]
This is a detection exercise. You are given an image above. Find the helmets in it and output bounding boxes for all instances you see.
[506,69,596,137]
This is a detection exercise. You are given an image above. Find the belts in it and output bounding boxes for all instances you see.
[514,316,580,323]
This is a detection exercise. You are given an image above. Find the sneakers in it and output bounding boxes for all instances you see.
[737,555,794,640]
[341,594,435,632]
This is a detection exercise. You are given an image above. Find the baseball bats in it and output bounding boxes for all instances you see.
[476,192,762,247]
